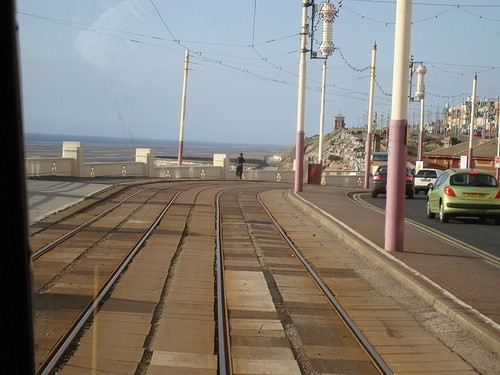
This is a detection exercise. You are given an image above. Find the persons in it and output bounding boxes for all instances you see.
[234,153,245,180]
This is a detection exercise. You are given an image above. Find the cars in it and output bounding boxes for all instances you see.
[414,169,438,196]
[371,164,414,199]
[426,168,500,223]
